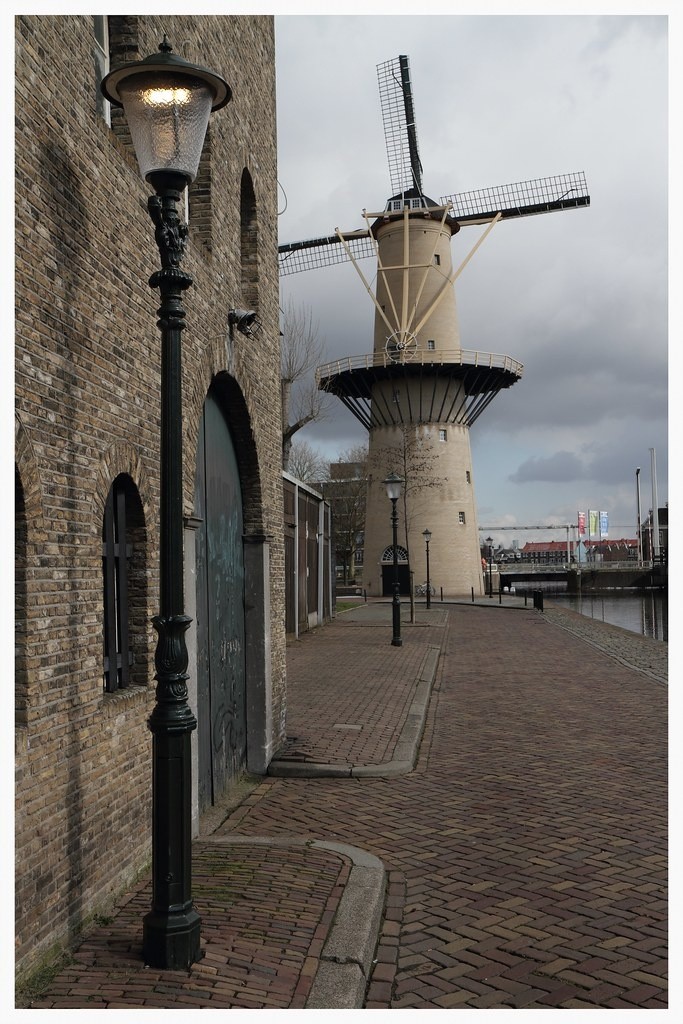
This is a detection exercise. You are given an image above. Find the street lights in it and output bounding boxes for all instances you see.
[485,535,494,599]
[101,32,234,975]
[380,471,406,647]
[421,528,433,609]
[636,466,641,568]
